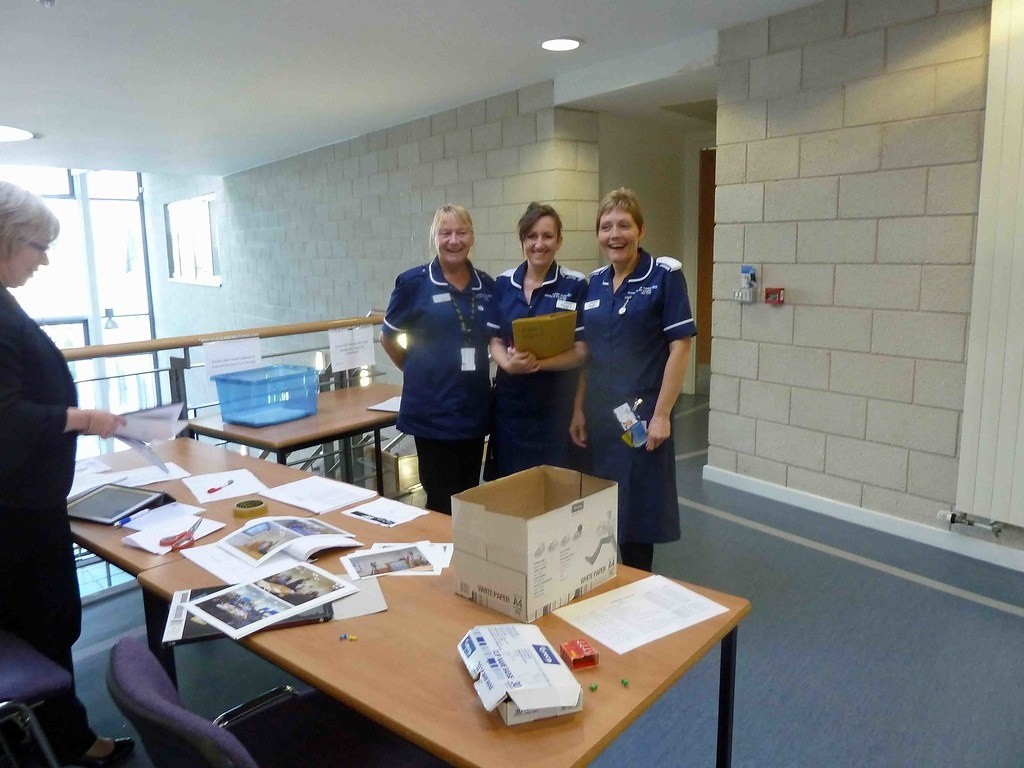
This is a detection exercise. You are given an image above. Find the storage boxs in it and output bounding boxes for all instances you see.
[211,364,319,428]
[456,623,583,726]
[450,464,618,623]
[364,444,427,510]
[561,638,600,670]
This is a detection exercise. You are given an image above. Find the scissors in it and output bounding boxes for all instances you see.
[159,518,204,551]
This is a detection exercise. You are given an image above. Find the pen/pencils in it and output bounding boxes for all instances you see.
[113,507,150,528]
[207,480,233,494]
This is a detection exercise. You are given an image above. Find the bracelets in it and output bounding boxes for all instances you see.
[83,408,92,436]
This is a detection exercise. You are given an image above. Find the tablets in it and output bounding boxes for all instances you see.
[67,483,162,524]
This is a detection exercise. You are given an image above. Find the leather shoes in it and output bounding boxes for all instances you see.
[71,736,135,768]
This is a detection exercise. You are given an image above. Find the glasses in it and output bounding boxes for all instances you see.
[21,238,49,255]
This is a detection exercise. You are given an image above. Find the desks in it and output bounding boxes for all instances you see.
[67,437,382,577]
[186,381,423,501]
[137,496,751,768]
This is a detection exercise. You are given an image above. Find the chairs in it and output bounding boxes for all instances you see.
[106,634,451,768]
[0,627,71,768]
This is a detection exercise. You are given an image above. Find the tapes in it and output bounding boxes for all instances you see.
[233,498,268,519]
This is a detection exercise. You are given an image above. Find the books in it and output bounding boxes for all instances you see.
[181,516,365,586]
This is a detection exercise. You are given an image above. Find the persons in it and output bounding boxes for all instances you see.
[569,186,699,573]
[0,182,135,767]
[381,204,495,516]
[489,202,589,481]
